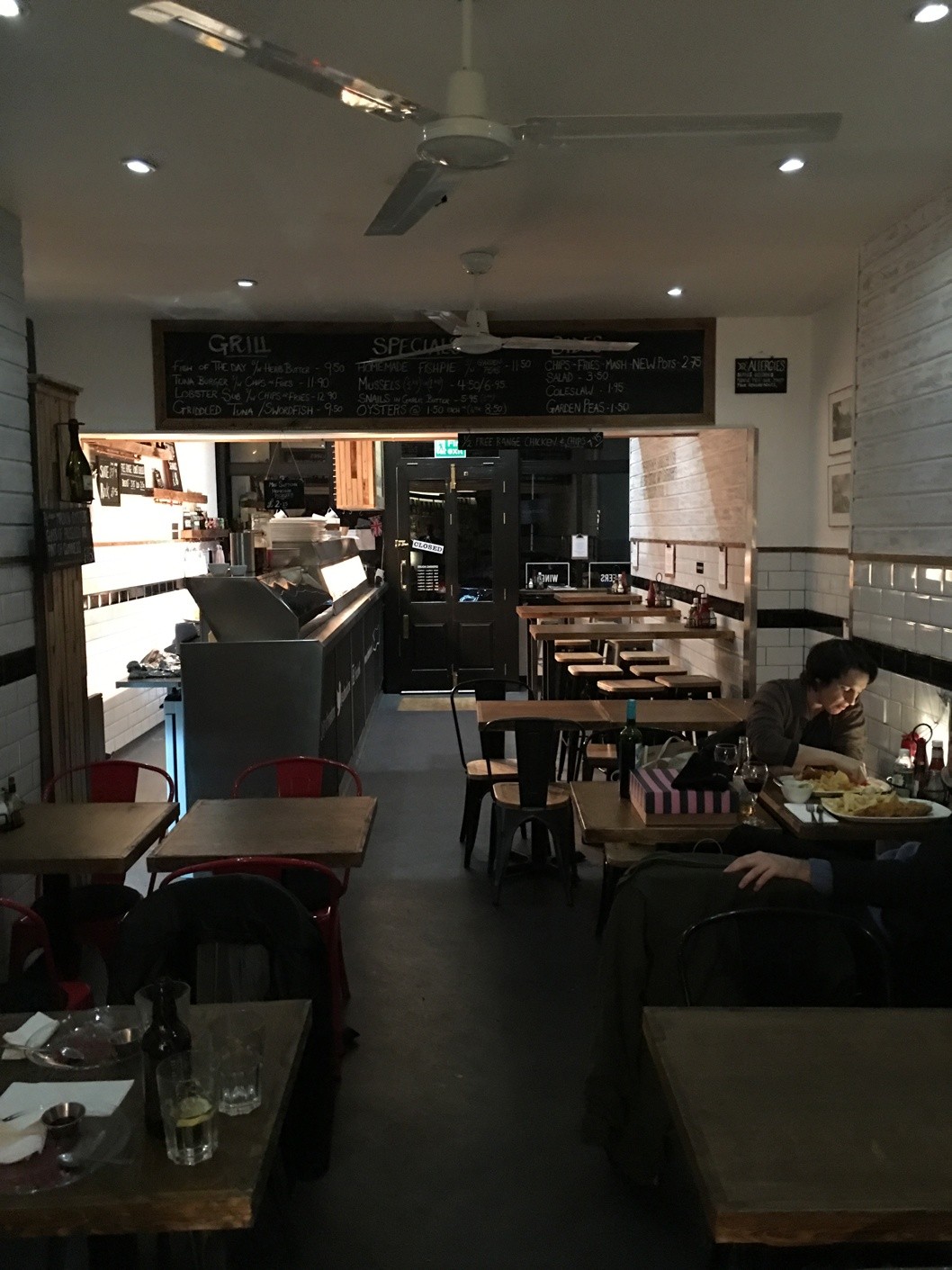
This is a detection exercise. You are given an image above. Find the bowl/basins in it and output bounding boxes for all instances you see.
[230,566,247,576]
[268,548,299,567]
[208,564,230,575]
[781,781,813,804]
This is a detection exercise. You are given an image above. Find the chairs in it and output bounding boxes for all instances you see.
[0,754,363,1016]
[446,682,892,1190]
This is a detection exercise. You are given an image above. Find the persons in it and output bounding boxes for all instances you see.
[720,811,952,908]
[703,638,870,788]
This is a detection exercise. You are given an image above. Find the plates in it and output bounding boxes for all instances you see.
[25,1004,152,1070]
[778,775,952,823]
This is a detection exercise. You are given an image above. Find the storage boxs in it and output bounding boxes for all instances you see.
[627,768,740,814]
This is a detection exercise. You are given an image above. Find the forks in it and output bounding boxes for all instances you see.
[805,804,817,824]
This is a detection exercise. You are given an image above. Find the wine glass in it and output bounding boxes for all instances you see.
[741,762,768,826]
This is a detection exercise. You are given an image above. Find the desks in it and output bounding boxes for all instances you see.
[555,593,642,603]
[528,623,736,700]
[0,996,314,1270]
[516,603,682,697]
[475,700,769,858]
[569,780,780,947]
[0,800,181,905]
[642,1004,951,1270]
[748,774,951,850]
[148,796,381,867]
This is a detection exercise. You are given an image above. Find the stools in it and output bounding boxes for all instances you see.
[539,619,723,753]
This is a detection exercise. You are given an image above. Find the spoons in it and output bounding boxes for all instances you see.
[817,805,824,823]
[1,1043,84,1060]
[57,1130,105,1166]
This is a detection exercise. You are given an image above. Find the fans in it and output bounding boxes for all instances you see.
[130,3,843,234]
[352,248,640,366]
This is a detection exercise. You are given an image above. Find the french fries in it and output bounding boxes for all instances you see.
[810,772,845,791]
[824,787,896,811]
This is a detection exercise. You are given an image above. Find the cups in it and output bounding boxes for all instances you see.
[715,744,740,773]
[207,1009,267,1116]
[155,1049,223,1166]
[135,982,190,1041]
[41,1102,85,1139]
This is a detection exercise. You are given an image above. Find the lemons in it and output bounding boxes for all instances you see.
[177,1097,215,1127]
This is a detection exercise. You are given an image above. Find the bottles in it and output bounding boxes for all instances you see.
[9,783,22,813]
[709,608,716,624]
[733,736,757,816]
[619,699,644,798]
[142,980,191,1132]
[528,578,533,589]
[689,598,699,626]
[243,530,267,572]
[536,572,543,589]
[647,581,655,607]
[660,591,666,606]
[698,594,709,626]
[617,574,624,593]
[67,418,93,502]
[0,791,9,825]
[891,738,945,805]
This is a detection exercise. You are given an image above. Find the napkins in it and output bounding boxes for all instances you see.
[2,1011,59,1060]
[0,1079,135,1164]
[784,803,839,823]
